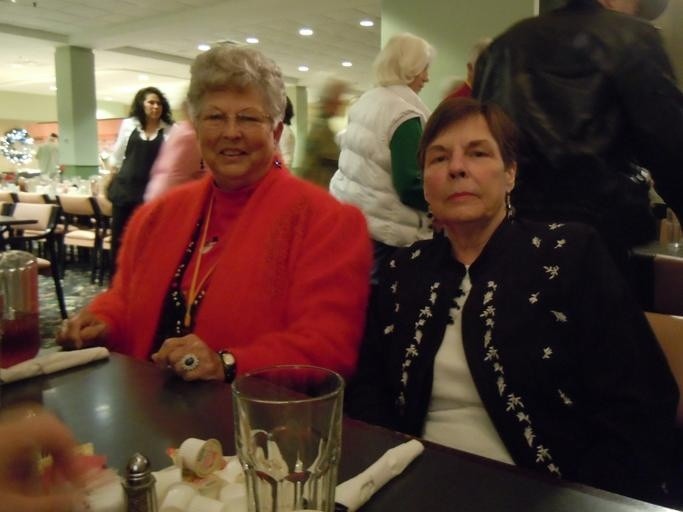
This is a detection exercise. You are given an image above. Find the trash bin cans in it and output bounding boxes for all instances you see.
[217,351,236,384]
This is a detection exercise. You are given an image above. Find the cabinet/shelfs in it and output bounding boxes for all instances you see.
[122,452,157,512]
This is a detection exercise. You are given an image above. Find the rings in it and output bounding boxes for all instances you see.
[181,354,196,370]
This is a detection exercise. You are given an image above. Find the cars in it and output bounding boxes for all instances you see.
[90,175,102,196]
[231,365,344,512]
[1,250,39,368]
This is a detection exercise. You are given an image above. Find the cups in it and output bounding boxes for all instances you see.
[194,110,275,128]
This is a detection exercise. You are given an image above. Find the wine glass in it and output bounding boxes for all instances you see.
[181,354,196,370]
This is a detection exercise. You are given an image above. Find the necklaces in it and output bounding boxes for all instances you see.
[182,192,228,327]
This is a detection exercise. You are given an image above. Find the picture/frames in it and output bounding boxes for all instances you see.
[0,215,38,254]
[0,346,683,512]
[635,242,683,301]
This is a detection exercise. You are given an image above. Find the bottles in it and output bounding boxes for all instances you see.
[605,0,667,21]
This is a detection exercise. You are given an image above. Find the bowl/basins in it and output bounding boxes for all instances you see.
[182,192,228,327]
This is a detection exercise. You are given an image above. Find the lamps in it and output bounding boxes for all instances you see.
[55,193,106,283]
[0,190,17,206]
[0,202,68,321]
[12,191,80,279]
[92,193,114,286]
[642,310,683,390]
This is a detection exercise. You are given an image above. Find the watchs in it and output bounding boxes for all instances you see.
[217,351,236,384]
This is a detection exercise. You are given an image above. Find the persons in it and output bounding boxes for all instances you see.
[56,42,372,386]
[105,87,174,274]
[1,401,92,511]
[326,98,683,511]
[445,37,492,99]
[328,34,435,284]
[297,77,353,189]
[473,1,683,310]
[143,104,195,201]
